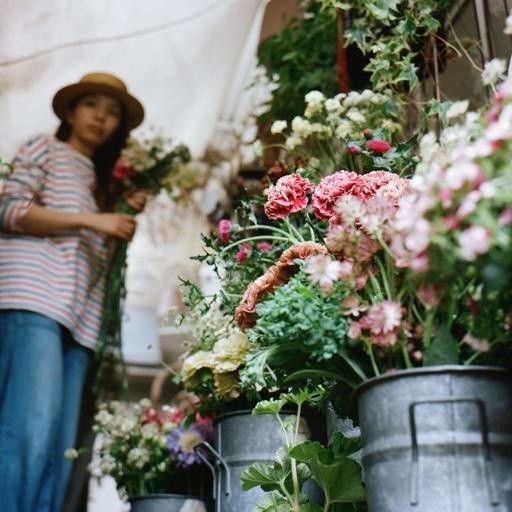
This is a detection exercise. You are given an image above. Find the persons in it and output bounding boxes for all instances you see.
[0,74,147,512]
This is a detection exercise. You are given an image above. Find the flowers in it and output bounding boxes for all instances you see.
[85,133,198,396]
[63,397,216,494]
[161,271,322,413]
[232,164,512,376]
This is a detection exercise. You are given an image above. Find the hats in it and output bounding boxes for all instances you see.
[52,72,144,132]
